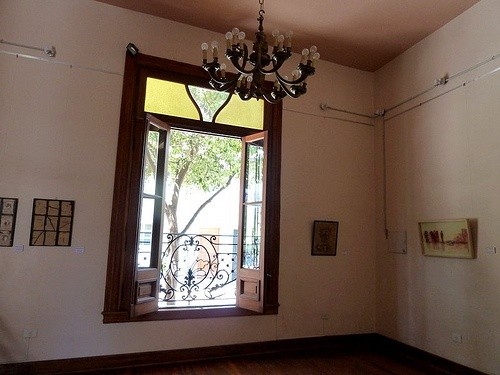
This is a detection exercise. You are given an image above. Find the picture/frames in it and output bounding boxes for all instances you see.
[29,197,75,247]
[0,197,18,247]
[418,217,475,259]
[311,220,339,256]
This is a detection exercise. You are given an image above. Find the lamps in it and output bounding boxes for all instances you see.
[201,0,321,105]
